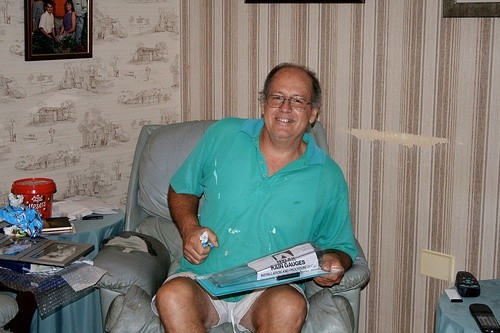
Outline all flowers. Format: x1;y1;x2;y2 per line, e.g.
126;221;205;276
0;193;44;239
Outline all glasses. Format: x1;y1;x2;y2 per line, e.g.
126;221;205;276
265;94;313;111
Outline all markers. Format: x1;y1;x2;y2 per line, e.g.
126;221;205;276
82;216;104;220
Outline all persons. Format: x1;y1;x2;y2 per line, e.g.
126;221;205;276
33;0;87;53
151;64;360;333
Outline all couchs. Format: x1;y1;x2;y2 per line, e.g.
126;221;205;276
91;120;370;333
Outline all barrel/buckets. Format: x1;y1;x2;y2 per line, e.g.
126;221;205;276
10;177;56;218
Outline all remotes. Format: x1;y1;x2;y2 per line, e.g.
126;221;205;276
456;271;480;298
470;304;500;333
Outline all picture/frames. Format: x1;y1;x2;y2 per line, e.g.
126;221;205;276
443;0;500;17
24;0;93;61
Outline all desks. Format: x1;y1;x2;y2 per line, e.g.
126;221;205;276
31;209;126;333
434;279;500;333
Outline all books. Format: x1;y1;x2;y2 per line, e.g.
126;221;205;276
41;216;73;235
